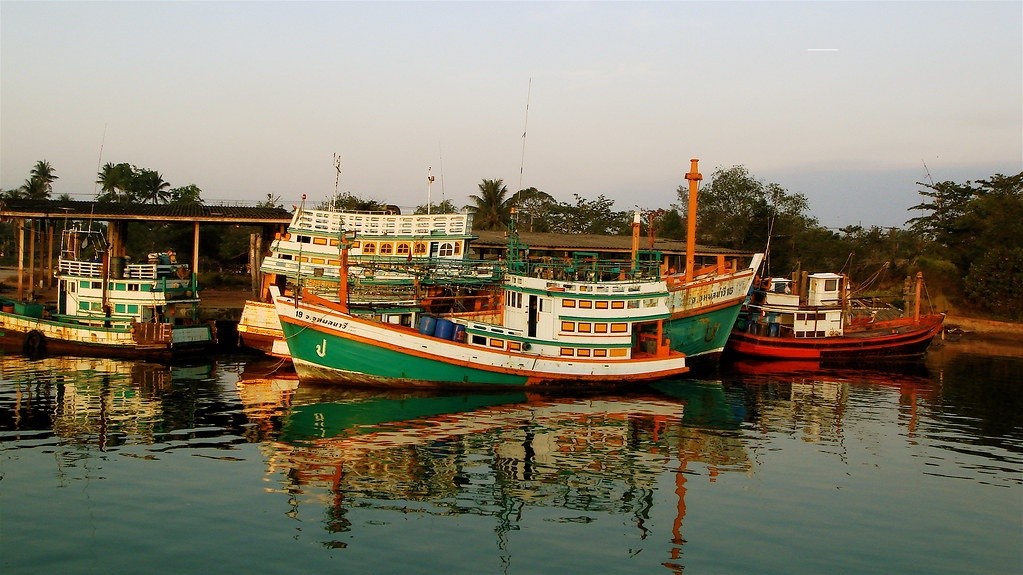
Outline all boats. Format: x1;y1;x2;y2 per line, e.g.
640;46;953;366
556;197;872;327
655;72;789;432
0;122;219;358
262;374;683;535
269;213;690;388
453;157;764;368
727;248;950;374
235;152;513;361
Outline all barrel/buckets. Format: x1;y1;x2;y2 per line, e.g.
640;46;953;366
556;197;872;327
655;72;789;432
768;323;780;338
419;314;437;336
111;256;125;280
434;318;454;340
756;322;769;337
3;303;15;314
451;324;465;344
744;320;756;336
